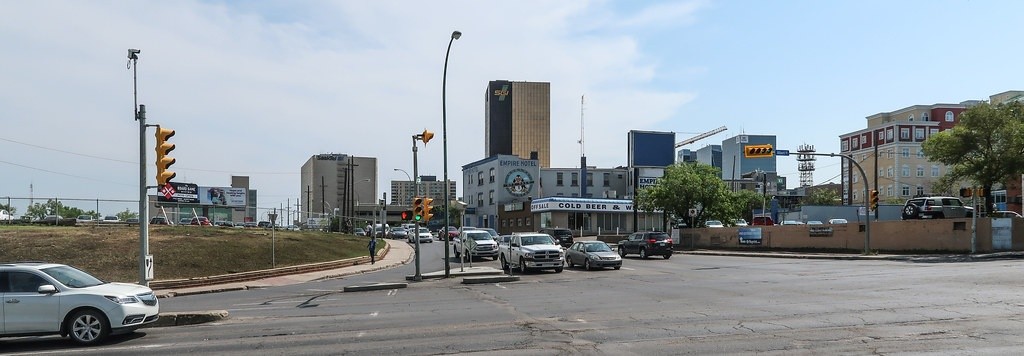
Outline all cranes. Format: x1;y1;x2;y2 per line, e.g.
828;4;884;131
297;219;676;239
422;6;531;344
675;126;727;150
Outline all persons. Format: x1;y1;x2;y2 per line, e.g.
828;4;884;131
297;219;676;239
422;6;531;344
991;202;997;211
365;222;372;237
472;234;477;240
587;245;593;252
422;229;427;233
596;245;604;251
367;236;375;265
39;278;49;285
539;238;545;243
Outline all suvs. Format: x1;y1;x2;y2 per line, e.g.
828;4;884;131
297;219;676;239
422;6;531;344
901;193;973;219
538;228;574;248
453;230;564;273
618;231;674;260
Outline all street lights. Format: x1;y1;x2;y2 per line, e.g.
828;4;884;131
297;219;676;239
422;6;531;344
394;168;412;206
441;30;462;276
323;179;371;218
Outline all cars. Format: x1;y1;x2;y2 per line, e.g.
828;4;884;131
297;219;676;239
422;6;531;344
0;207;299;229
352;223;476;243
704;215;852;228
565;241;623;270
0;262;161;346
993;211;1023;217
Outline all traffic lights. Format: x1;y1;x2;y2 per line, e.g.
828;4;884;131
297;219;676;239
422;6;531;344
744;145;773;157
402;197;434;223
155;127;176;186
